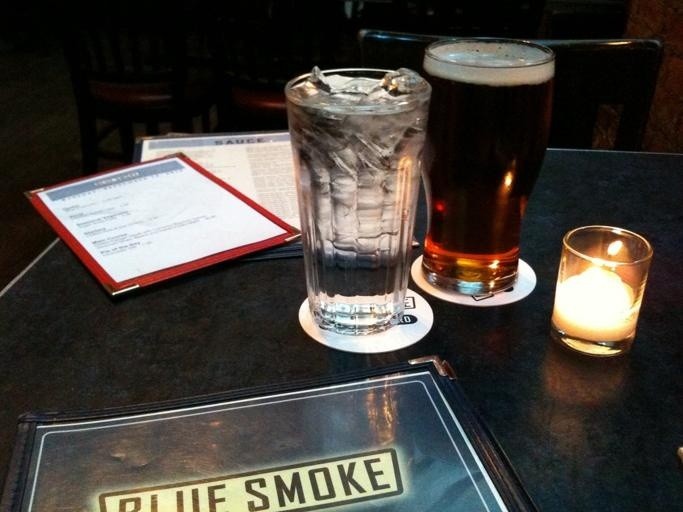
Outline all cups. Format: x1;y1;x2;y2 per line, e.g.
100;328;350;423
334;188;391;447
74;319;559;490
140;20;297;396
418;37;555;297
550;226;653;356
288;65;430;336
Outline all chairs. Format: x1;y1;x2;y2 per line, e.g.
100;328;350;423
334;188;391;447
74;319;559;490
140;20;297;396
358;31;665;155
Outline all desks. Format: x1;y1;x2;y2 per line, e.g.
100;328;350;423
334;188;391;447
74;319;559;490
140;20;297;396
2;148;683;510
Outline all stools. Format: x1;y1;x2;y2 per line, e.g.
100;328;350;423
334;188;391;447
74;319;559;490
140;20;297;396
82;78;291;172
